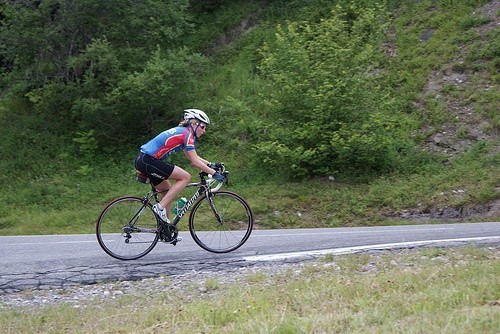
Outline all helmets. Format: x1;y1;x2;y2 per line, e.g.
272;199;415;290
184;109;210;125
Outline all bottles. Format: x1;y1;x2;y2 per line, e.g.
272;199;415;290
172;197;188;214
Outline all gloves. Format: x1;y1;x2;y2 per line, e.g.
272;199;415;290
212;172;225;183
208;162;224;171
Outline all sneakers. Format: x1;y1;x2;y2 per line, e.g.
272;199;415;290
152;203;170;223
171;233;182;240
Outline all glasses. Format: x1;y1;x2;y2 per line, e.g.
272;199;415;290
195;122;206;129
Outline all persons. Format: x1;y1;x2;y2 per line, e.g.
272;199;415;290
134;109;226;244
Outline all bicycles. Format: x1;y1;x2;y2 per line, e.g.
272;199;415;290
96;161;254;261
